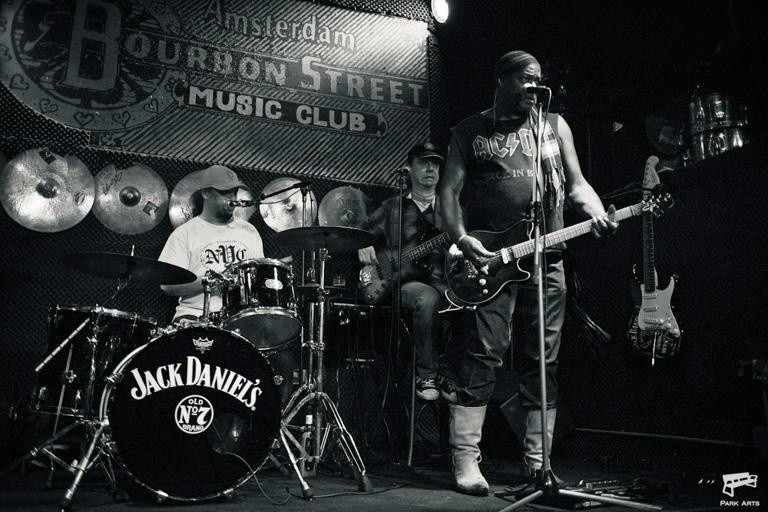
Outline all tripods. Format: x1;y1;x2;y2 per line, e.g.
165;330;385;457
381;189;436;489
496;105;661;511
262;193;371;495
19;326;129;512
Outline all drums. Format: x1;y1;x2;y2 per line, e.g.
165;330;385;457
222;258;302;353
33;306;167;419
682;85;756;166
100;321;282;503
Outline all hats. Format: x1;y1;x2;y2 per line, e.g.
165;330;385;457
200;165;249;194
408;141;445;166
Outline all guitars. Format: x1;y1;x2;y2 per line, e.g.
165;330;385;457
627;155;683;365
345;230;450;304
443;184;673;304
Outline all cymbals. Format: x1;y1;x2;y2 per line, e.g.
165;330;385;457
317;186;375;231
63;253;197;285
170;173;255;230
275;227;378;249
260;176;318;234
94;163;168;235
1;147;95;233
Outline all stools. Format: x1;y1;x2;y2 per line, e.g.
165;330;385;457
381;307;449;465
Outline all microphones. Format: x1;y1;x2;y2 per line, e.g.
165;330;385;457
519;82;550;101
226;198;266;212
395;163;414;184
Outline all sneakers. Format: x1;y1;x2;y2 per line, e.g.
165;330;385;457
416;373;458;403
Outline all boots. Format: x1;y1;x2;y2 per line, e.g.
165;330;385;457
522;405;564;483
447;403;491;494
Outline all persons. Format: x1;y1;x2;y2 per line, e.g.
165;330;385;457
156;165;267;326
440;51;620;496
355;144;459;404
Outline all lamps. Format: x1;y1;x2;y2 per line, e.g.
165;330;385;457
432;0;449;23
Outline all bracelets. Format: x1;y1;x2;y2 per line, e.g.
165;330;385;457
455;234;466;247
